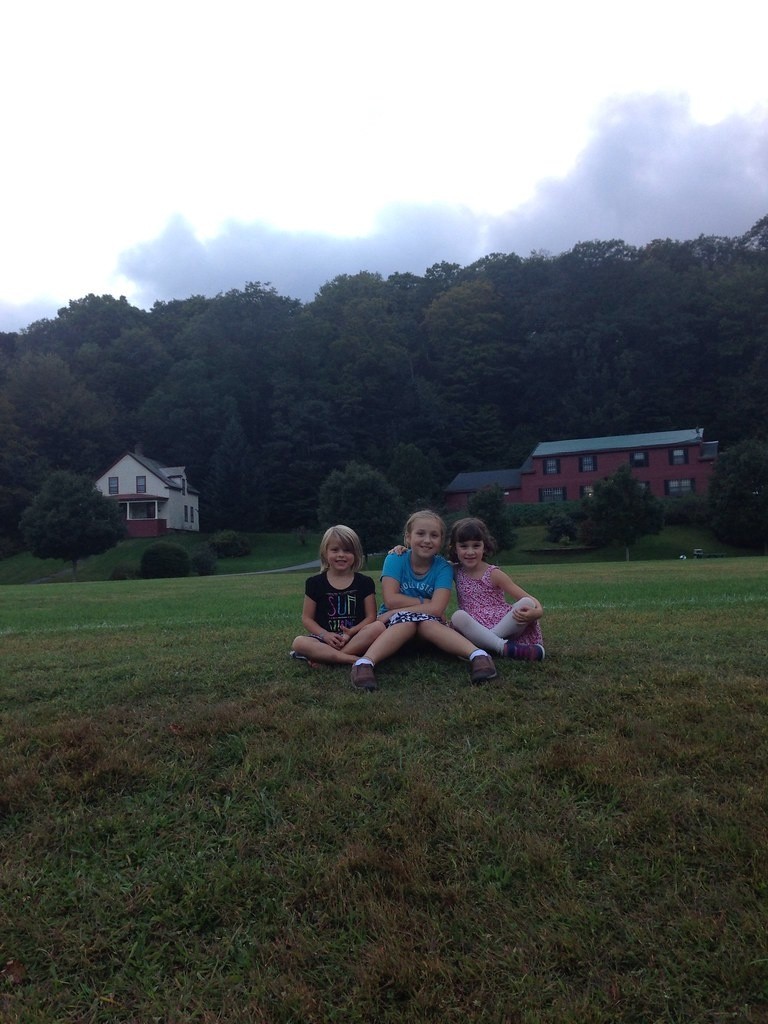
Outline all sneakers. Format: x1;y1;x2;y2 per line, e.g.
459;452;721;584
470;654;499;684
505;642;546;661
351;663;378;692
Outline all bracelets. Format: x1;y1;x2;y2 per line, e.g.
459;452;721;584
319;632;324;638
419;597;423;604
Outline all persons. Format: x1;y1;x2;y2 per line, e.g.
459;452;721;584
388;518;545;662
350;509;496;691
289;525;386;669
